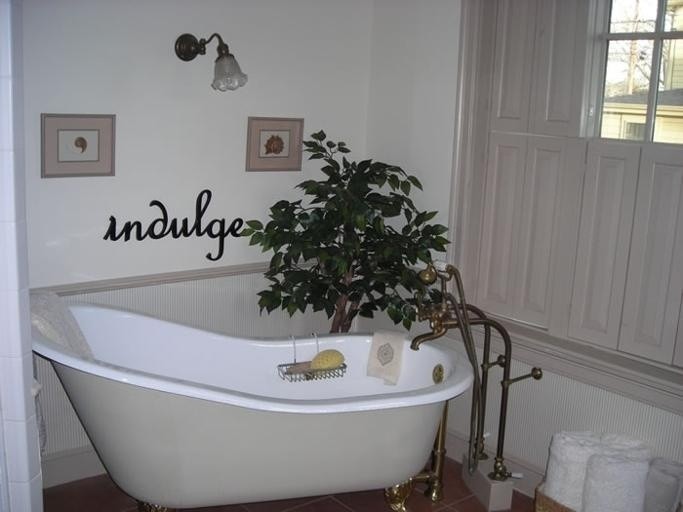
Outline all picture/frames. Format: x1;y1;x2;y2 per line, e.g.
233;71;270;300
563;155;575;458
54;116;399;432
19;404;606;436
40;114;116;178
246;116;305;171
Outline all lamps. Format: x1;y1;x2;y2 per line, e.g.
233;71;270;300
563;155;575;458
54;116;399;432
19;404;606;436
174;32;248;92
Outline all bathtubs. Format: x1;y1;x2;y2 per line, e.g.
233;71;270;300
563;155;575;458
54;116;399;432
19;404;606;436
32;303;474;512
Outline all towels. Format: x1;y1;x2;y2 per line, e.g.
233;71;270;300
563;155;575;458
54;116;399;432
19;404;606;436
366;331;405;385
541;430;683;511
29;290;95;450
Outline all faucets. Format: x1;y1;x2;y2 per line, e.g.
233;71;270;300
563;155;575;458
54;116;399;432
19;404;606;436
410;327;447;350
